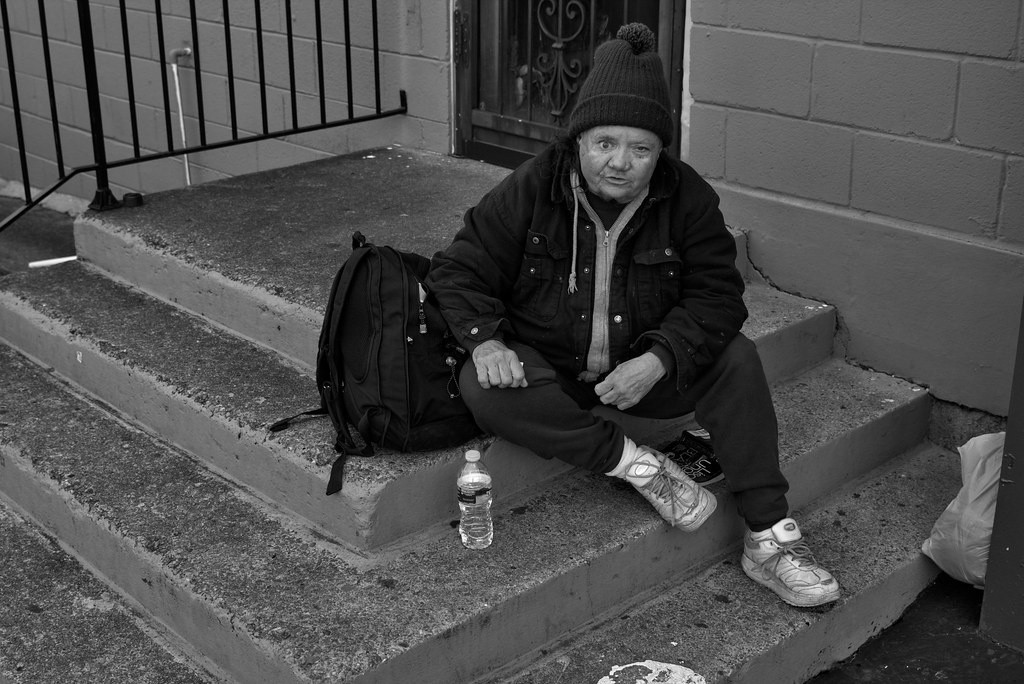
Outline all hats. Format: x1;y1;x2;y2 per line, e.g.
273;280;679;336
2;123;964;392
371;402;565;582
568;22;674;146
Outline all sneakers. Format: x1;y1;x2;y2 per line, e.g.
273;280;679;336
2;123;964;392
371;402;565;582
741;518;840;607
624;445;717;533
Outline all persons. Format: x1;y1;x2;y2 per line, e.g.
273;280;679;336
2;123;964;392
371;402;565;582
426;22;840;608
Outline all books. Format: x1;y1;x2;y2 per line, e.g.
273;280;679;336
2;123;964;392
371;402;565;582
660;427;726;487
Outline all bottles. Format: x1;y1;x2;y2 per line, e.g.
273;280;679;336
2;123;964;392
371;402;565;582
457;450;495;549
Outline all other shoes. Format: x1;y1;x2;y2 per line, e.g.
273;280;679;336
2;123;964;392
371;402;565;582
660;427;725;487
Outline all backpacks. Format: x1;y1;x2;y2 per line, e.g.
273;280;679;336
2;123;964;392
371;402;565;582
316;231;482;457
